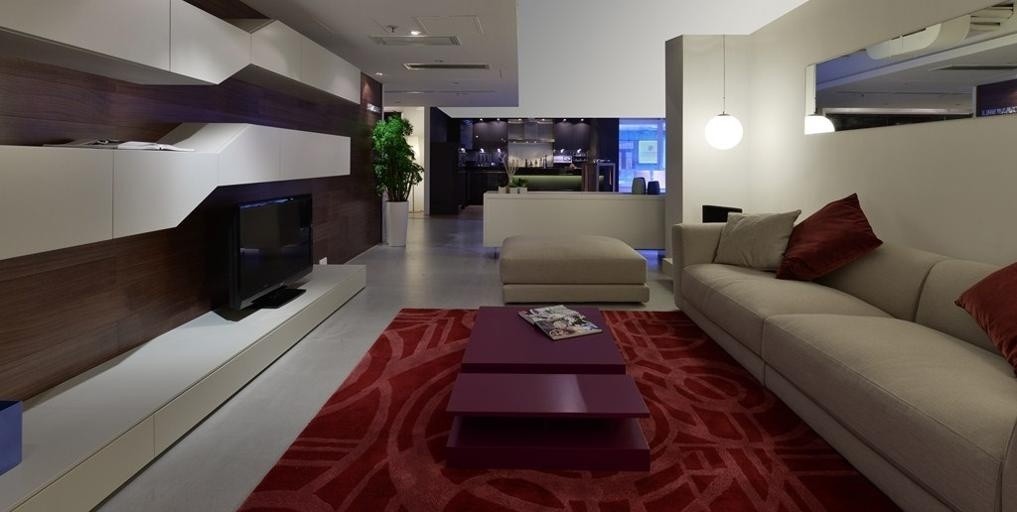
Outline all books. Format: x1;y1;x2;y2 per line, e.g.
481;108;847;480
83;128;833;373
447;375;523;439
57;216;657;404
518;305;603;340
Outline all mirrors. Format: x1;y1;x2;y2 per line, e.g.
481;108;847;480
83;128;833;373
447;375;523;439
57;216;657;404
799;0;1016;138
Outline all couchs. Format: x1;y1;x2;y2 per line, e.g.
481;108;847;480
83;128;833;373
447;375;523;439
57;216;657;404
499;234;650;304
669;217;1017;510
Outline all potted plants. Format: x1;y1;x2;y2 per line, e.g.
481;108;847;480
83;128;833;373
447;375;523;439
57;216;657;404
366;110;424;249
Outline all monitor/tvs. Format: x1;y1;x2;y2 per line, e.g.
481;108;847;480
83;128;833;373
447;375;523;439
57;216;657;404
226;194;314;310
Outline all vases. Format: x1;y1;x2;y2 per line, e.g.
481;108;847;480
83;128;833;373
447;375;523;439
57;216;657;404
646;180;662;196
630;175;648;195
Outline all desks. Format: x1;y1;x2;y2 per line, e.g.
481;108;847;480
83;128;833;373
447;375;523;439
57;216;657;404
0;264;367;511
481;190;666;262
444;305;652;474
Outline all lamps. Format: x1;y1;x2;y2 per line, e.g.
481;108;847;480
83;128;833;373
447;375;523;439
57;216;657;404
803;85;837;135
705;34;745;154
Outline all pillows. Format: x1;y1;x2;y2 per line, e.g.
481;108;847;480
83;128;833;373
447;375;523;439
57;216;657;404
774;191;886;282
952;260;1016;379
711;208;802;272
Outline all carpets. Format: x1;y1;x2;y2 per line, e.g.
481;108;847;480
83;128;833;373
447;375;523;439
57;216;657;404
233;305;902;510
